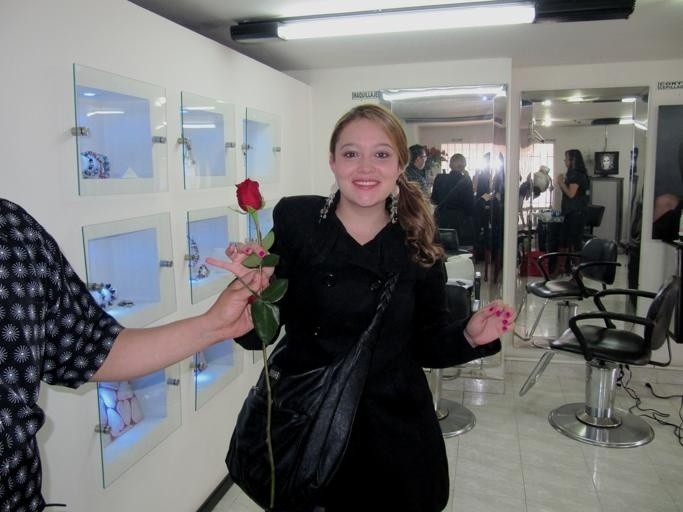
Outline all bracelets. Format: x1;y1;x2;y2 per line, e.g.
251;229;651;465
187;238;209;281
92;283;116;307
86;286;105;308
78;152;110;179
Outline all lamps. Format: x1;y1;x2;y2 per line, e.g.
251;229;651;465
229;0;636;44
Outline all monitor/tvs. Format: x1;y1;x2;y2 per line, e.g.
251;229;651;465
595;152;618;178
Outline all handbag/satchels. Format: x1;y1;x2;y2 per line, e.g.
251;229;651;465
226;342;368;511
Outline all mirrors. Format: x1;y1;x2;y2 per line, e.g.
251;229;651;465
380;83;506;310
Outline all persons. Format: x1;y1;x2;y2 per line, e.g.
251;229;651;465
0;197;272;512
599;154;613;170
203;103;518;511
555;148;590;255
402;142;505;268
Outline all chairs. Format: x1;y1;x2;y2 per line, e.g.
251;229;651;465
423;366;477;438
507;236;622;343
574;204;605;249
519;274;682;448
437;229;476;259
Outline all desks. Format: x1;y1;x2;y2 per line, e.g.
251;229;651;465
537;218;566;270
590;177;623;249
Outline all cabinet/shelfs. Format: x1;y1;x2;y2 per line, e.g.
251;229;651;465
100;362;170;460
190;218;233;288
76;87;158;179
179;108;227;178
86;226;162;318
194;343;236;385
247;204;278;259
244;117;276;180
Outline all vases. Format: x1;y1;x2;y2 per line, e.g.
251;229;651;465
424;169;436;185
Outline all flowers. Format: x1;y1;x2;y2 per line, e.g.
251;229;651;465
420;143;450;171
230;177;292;508
